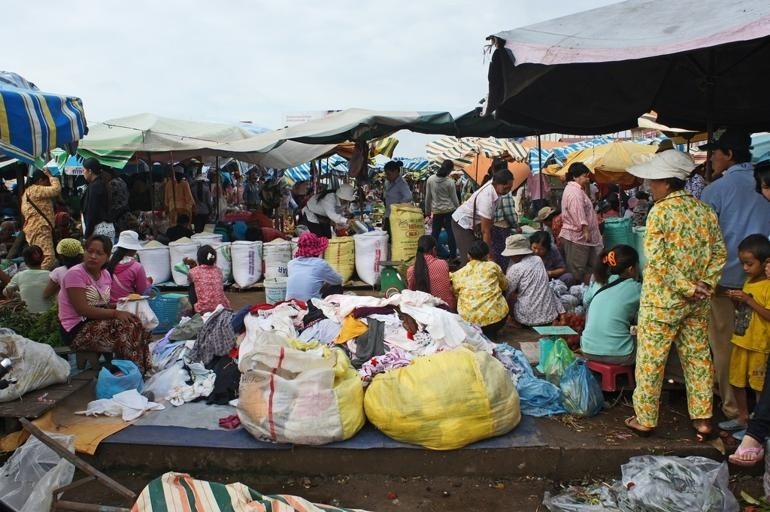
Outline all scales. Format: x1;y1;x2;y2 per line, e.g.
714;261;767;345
376;260;408;292
532;325;578;378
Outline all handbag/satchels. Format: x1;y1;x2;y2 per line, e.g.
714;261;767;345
474;224;482;241
144;286;180;334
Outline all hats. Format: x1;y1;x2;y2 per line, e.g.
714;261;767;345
625;148;696;181
293;233;329;258
56;237;84;258
337;184;356;202
113;230;144;251
655;139;674;154
698;128;752;150
532;206;556;223
500;234;533;257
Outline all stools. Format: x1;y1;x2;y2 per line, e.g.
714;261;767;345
584;361;633;393
75;350;114;370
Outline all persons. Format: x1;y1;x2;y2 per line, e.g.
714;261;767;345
0;125;770;469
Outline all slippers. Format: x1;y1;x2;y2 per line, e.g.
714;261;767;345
718;418;766;467
625;416;651;438
691;419;714;441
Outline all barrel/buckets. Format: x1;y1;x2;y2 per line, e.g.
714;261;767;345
263;276;288;306
